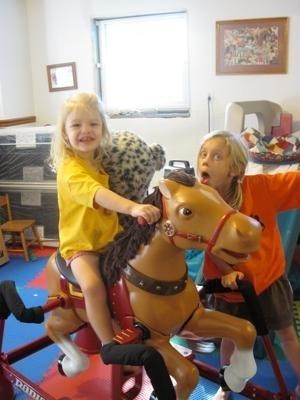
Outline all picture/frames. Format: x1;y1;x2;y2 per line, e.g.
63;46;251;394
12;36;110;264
215;16;290;77
46;62;78;92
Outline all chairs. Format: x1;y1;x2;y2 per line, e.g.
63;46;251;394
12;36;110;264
0;194;44;262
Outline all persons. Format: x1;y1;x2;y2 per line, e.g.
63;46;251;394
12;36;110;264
42;91;163;373
195;130;300;400
